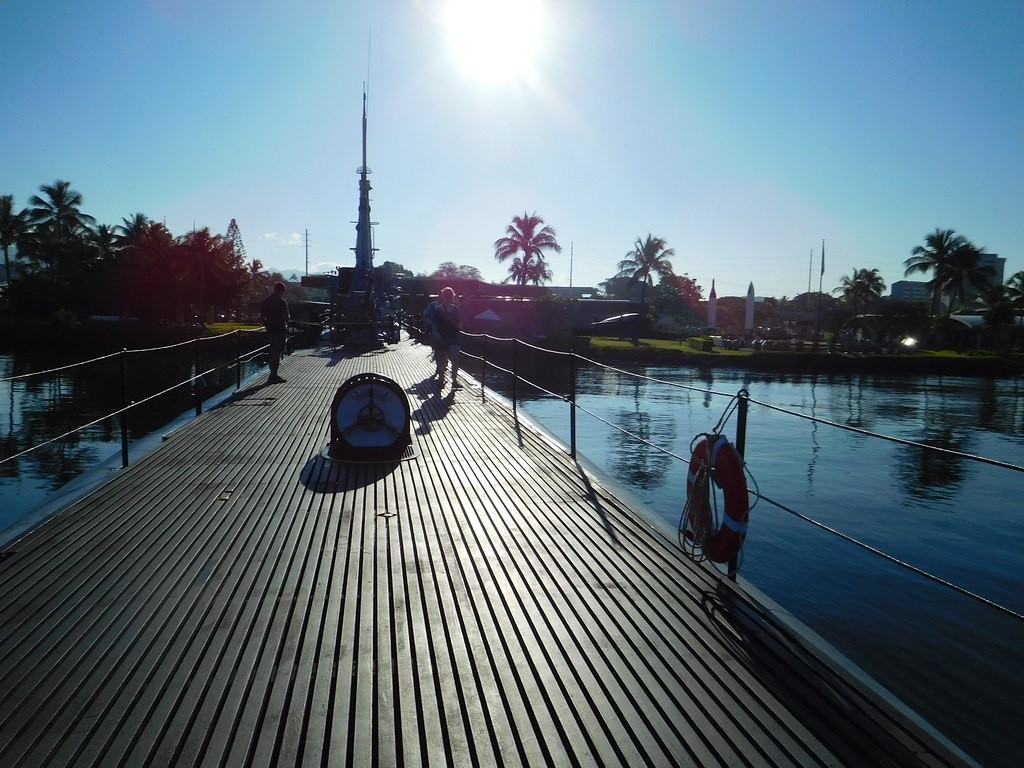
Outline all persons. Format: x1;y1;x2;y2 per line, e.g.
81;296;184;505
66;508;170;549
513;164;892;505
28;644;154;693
424;287;463;389
262;282;291;384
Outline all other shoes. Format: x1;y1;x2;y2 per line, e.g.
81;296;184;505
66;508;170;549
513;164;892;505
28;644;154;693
437;380;444;389
452;382;463;388
268;375;286;384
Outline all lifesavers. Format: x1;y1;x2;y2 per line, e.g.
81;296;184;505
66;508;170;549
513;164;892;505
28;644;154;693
685;434;751;563
